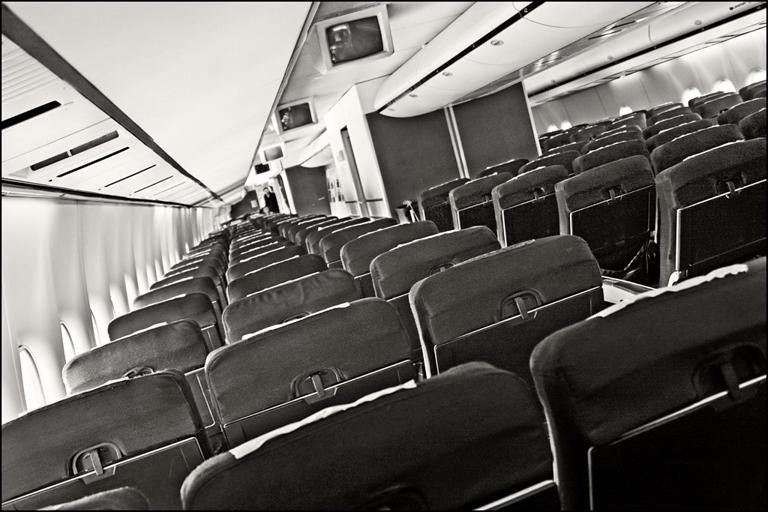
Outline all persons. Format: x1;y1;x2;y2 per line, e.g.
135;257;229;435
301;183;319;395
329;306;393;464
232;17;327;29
261;187;280;214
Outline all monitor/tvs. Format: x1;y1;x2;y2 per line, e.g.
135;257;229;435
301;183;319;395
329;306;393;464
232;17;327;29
253;163;271;176
258;141;287;166
273;98;319;136
316;3;394;75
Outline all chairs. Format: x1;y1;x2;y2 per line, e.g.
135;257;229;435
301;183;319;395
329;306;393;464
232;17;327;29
553;153;660;289
570;124;599;144
648;102;683;118
172;235;223;265
480;158;529;180
1;367;215;511
645;118;715;153
717;96;767;124
221;269;362;345
692;92;732;106
539;130;562;148
227;222;274;238
542;131;574;147
449;172;513;237
179;360;561;512
149;266;227;309
257;211;288;229
739;79;767;102
61;318;209;399
538;139;588;153
318;216;398;270
204;296;412;445
416;175;472;231
647;107;692;128
107;292;224;351
688;90;723;107
607;114;646;130
518;150;580;171
305;216;370;255
227;240;293;268
643;112;703;140
224;244;308;285
694;94;743;119
295;215;351;247
36;485;152;511
339;219;438;278
225;253;328;306
593;124;642;138
288;215;328;244
490;163;569;248
739;107;768;138
580;130;640;155
614;113;647;118
650;123;744;175
529;254;767;510
368;225;502;366
408;233;603;376
132;276;225;344
570;138;648;174
654;136;768;289
